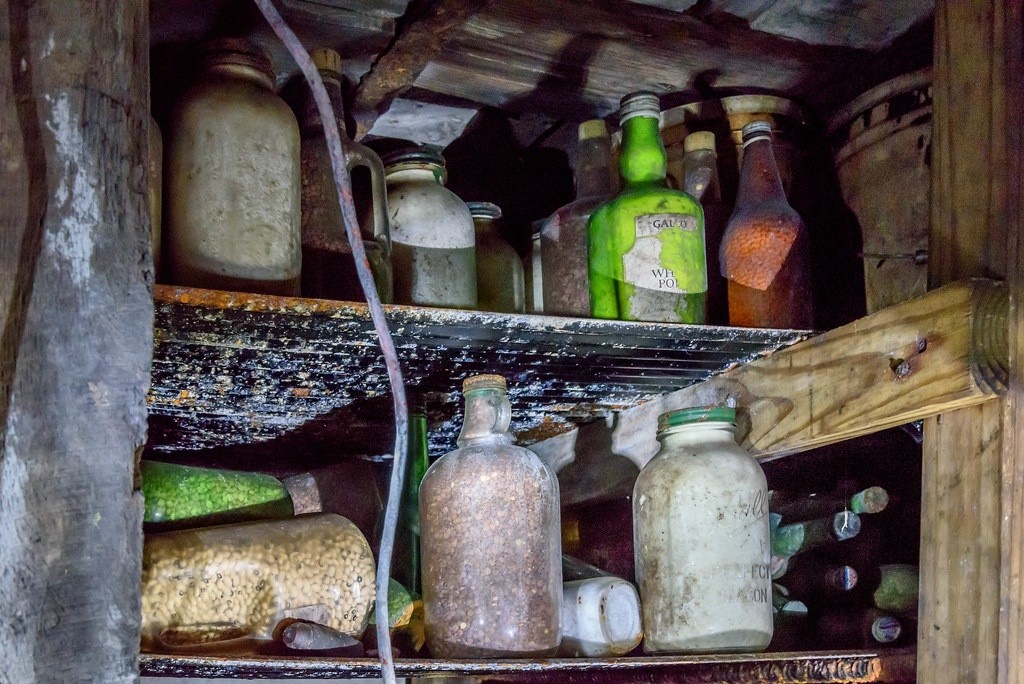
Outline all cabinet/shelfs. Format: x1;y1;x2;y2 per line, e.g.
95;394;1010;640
0;0;1024;684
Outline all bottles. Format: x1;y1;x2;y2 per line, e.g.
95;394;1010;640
134;37;904;656
